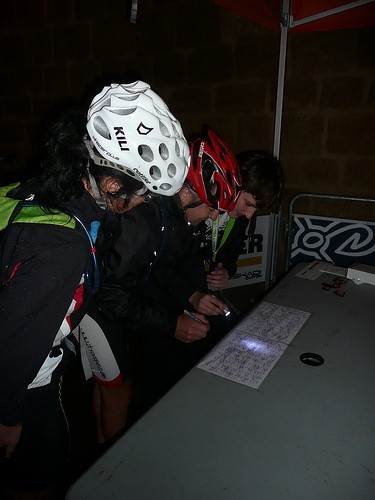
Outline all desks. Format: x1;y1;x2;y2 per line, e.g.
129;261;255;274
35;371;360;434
66;260;375;500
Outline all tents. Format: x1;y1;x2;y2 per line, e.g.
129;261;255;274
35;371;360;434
211;1;375;291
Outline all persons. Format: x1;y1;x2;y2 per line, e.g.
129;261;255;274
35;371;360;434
181;150;284;363
87;123;244;462
1;80;191;500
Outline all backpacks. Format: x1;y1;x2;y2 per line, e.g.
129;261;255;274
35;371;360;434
0;173;100;288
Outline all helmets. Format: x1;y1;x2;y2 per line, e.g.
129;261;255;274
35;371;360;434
81;79;192;195
184;127;246;214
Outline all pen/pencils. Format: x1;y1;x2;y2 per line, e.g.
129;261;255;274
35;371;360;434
183;309;209;327
